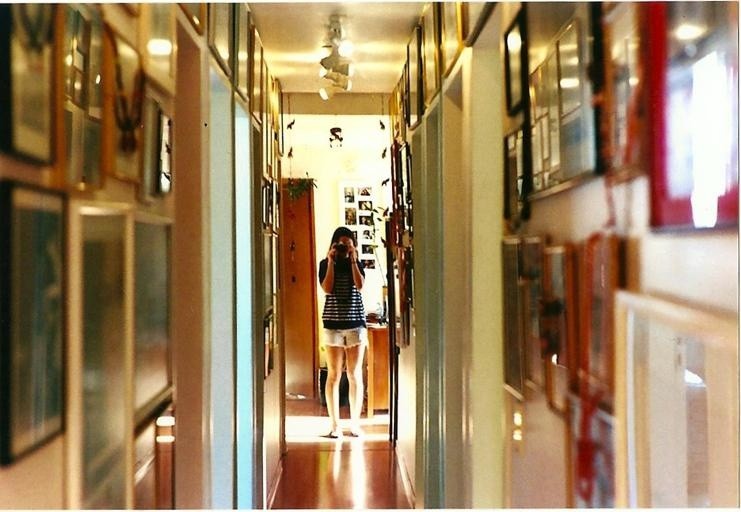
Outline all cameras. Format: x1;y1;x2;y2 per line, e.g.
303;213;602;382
332;242;349;260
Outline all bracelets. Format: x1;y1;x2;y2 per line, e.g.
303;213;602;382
351;260;357;264
330;260;337;264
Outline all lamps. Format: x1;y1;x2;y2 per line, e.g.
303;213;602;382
318;13;355;102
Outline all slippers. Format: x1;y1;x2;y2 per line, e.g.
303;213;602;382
351;431;360;437
329;431;338;438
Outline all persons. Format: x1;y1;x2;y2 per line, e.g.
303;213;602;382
319;226;373;437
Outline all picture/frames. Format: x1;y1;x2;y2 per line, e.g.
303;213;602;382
394;2;737;507
4;3;283;508
